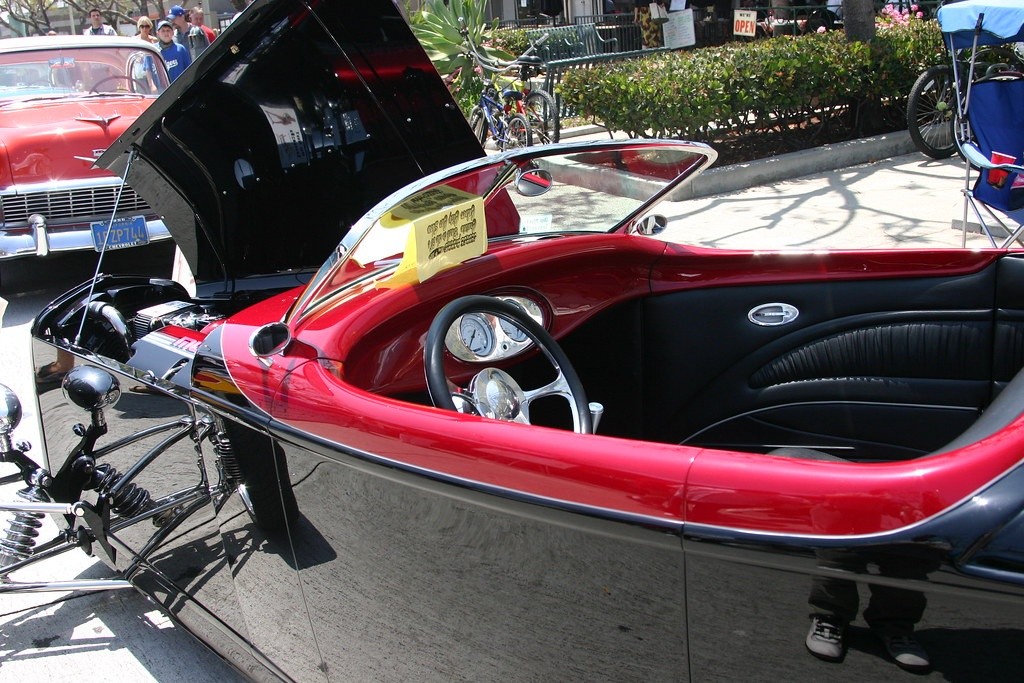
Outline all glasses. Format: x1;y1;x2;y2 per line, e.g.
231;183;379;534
139;25;150;29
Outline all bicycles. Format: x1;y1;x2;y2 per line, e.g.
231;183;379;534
453;15;560;153
905;47;1024;159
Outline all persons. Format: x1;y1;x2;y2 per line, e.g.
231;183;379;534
606;0;616;13
48;8;121;79
803;0;843;33
167;4;210;61
131;16;158;94
143;21;190;95
188;6;215;44
633;0;665;50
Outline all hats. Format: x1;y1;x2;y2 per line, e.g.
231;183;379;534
166;5;185;19
157;21;174;31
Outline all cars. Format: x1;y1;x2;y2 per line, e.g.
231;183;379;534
0;0;1024;683
0;35;176;295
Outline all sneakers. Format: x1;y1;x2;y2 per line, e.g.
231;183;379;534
806;617;846;658
884;633;931;667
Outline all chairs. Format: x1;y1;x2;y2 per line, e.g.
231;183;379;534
951;71;1024;248
651;257;1023;463
